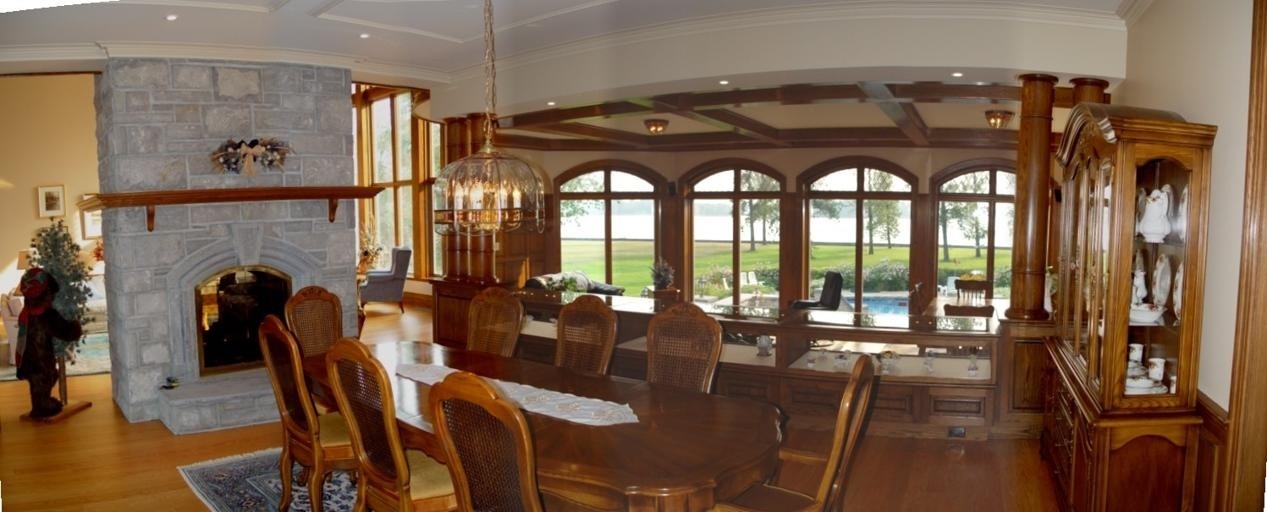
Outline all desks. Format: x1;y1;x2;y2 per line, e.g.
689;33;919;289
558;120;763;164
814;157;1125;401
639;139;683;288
921;293;1011;323
283;335;785;512
790;270;845;310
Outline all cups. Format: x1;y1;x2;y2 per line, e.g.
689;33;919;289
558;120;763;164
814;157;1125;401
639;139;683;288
654;298;664;311
605;296;612;306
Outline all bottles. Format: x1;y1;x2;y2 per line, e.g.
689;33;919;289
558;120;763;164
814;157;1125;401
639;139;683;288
756;334;772;356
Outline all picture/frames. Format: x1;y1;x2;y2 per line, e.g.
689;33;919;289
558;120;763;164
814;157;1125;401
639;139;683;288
80;192;102;240
36;184;66;220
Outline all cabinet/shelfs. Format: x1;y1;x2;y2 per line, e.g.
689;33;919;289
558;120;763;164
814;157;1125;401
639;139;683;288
1044;347;1204;511
1049;102;1220;416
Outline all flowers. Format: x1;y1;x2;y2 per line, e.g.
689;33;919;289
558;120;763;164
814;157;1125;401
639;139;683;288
651;259;674;287
210;136;293;176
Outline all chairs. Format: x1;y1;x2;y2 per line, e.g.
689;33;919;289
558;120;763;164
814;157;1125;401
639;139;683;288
427;368;547;512
945;305;997;318
283;285;347;415
458;285;525;356
707;352;878;510
247;315;358;511
360;245;412;313
326;338;459;512
953;278;993;298
553;293;620;376
646;302;722;394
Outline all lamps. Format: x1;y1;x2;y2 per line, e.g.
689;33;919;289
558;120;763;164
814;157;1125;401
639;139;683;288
424;1;553;242
643;119;669;136
985;109;1014;129
15;247;43;272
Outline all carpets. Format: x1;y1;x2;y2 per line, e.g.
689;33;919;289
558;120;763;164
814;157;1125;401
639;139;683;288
0;326;114;382
175;447;366;510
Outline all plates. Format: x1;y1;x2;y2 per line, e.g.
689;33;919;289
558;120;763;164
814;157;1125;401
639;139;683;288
1122;184;1188;397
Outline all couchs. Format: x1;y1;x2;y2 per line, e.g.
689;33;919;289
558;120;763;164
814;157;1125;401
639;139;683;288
528;268;624;296
1;259;24;365
